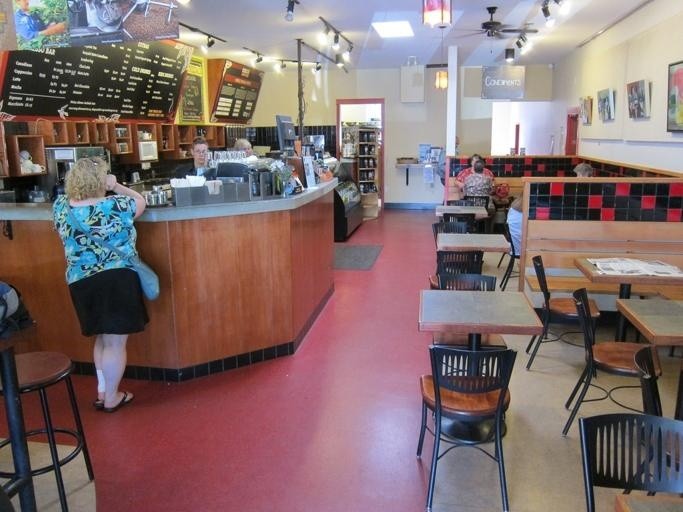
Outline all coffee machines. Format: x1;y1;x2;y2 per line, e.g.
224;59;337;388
38;146;104;199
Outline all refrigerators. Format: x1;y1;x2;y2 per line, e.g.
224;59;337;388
341;125;379;218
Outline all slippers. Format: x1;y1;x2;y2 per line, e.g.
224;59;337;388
93;391;135;413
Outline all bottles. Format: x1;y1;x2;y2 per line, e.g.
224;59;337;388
144;186;168;205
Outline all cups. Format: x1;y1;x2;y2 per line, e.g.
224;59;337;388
203;150;247;170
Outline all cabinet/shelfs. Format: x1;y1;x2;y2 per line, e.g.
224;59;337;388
357;126;378;207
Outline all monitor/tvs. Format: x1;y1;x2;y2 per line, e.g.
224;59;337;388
276;115;296;151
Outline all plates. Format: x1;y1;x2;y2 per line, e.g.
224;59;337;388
92;8;121;33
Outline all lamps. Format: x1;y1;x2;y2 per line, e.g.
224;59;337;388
205;35;216;48
422;1;450;90
255;52;264;64
331;33;354;68
285;1;299;23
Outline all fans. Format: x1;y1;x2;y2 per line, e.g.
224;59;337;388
456;6;538;40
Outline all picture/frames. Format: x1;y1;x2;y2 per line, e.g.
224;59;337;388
666;60;683;133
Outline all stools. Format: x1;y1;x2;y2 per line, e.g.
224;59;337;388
1;345;93;512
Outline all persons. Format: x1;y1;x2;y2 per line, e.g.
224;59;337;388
506;189;523;256
574;162;594;177
51;153;149;415
173;137;216;181
462;159;496;216
629;87;640;119
604;96;609;120
14;0;65;44
233;138;253;158
453;153;496;192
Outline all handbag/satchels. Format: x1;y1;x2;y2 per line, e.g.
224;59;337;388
495;183;510;197
130;253;160;300
